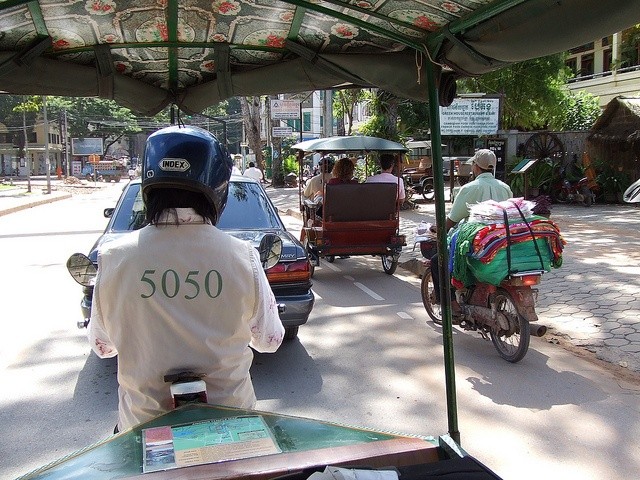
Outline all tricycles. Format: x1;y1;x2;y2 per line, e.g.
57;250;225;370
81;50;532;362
404;157;434;200
291;135;409;274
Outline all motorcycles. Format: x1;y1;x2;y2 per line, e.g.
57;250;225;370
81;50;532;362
552;170;592;206
413;220;548;363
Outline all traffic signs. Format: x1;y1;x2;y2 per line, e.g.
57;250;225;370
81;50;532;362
272;127;292;138
270;100;300;120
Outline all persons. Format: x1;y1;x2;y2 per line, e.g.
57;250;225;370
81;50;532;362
128;167;136;176
365;154;406;205
429;147;514;324
303;157;334;220
84;126;287;435
243;162;264;184
230;165;242;178
330;158;359;184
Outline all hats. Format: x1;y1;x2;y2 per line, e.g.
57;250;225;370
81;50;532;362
466;149;496;170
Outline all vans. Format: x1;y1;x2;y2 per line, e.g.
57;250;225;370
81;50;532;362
405;141;453;181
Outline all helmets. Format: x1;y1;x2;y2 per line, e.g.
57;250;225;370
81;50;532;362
141;124;230;225
319;158;335;174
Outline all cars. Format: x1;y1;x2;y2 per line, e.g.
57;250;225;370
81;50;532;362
77;175;314;339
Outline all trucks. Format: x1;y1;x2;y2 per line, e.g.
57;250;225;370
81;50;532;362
83;161;121;182
